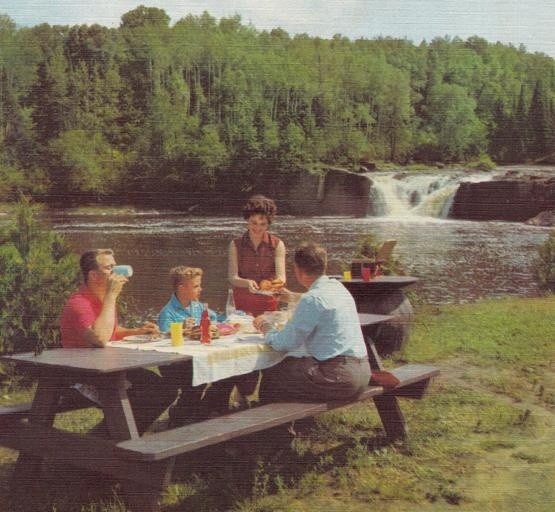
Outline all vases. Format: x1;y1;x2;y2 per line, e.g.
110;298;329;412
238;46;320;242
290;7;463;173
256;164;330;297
318;274;417;358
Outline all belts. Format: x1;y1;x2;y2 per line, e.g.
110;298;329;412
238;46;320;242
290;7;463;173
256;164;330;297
332;357;372;364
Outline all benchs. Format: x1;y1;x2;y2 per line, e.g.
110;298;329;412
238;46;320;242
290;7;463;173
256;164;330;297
1;398;97;421
114;364;440;512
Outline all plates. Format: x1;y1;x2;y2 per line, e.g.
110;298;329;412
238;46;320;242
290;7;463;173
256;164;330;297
123;334;163;343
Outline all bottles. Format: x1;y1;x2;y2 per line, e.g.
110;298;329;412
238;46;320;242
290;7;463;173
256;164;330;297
200;302;213;345
225;288;236;320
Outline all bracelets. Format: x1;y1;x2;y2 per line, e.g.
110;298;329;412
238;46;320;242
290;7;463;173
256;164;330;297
258;320;272;333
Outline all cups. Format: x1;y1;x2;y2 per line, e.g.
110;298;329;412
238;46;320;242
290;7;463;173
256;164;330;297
344;271;351;281
169;322;184;349
361;268;371;281
111;264;134;279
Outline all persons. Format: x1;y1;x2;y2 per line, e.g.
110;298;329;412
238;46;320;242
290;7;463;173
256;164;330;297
227;194;286;407
60;249;178;441
252;241;372;405
156;265;234;420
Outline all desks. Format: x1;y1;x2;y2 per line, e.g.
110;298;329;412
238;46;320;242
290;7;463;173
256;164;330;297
0;313;395;512
